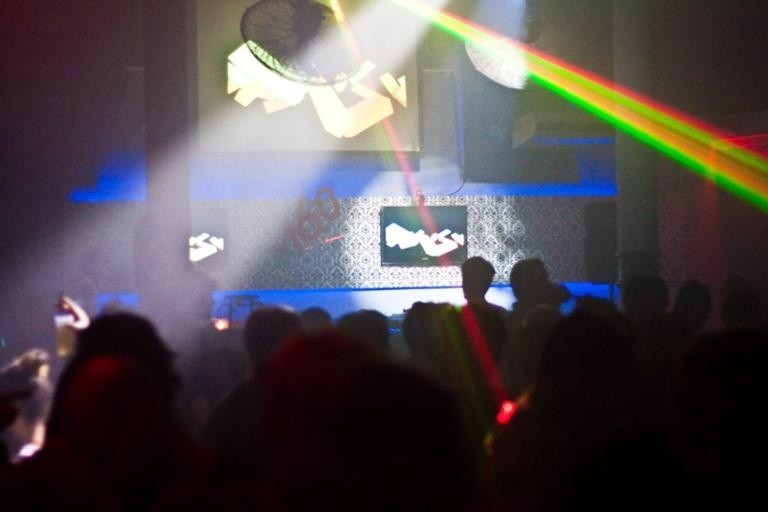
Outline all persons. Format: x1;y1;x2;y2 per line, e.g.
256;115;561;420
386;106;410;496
0;249;768;512
